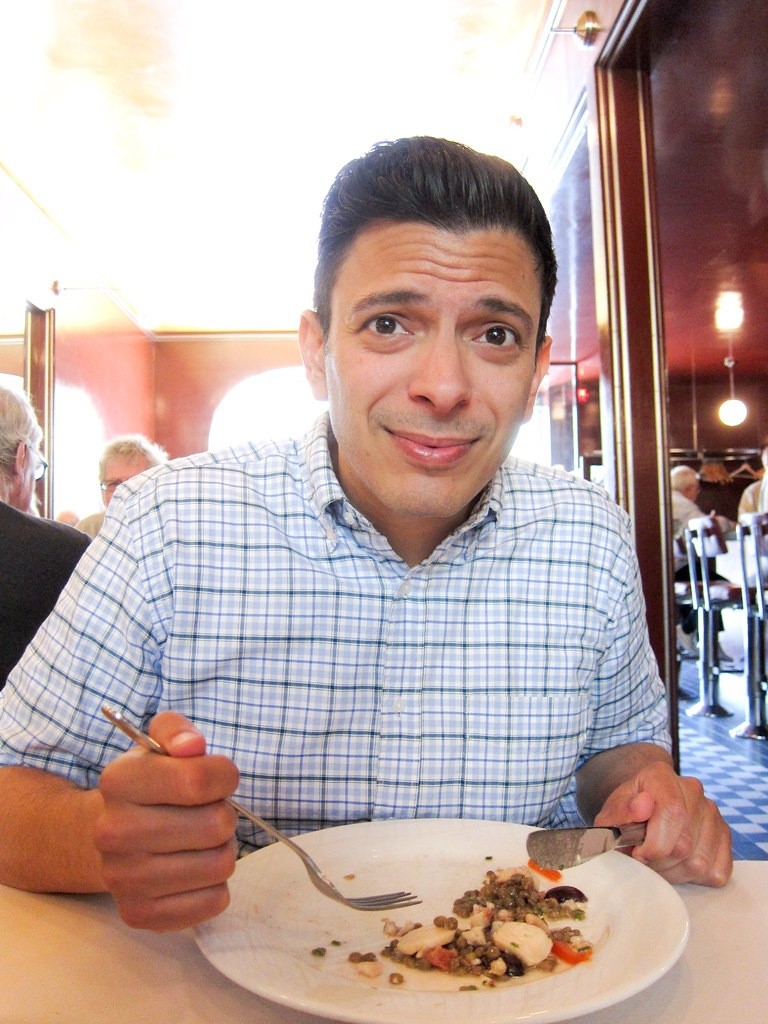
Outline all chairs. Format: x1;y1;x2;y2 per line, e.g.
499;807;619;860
674;512;768;743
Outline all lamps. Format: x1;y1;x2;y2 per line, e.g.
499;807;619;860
718;331;748;426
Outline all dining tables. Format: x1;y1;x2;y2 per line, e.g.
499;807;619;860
2;858;768;1024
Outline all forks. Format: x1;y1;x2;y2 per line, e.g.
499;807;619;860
95;702;424;913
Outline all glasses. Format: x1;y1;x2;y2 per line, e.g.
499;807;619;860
26;444;47;481
100;479;126;491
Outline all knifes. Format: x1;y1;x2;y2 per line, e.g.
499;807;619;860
524;820;645;875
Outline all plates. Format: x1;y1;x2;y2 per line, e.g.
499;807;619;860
186;816;691;1024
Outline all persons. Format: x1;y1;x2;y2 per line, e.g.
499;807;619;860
737;438;768;524
73;433;170;539
0;384;90;695
0;137;733;931
670;465;732;661
